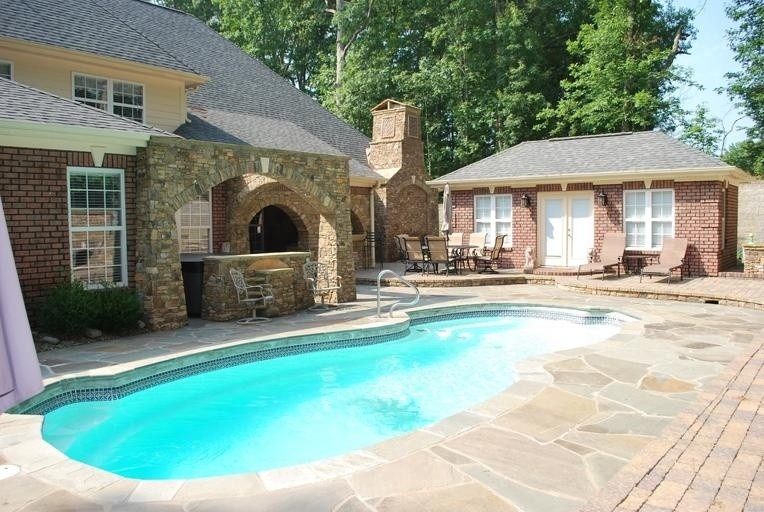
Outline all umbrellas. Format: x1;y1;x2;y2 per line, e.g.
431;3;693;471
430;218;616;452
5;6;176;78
0;192;46;418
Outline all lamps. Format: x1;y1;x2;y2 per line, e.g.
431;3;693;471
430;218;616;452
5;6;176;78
521;194;529;207
597;192;607;206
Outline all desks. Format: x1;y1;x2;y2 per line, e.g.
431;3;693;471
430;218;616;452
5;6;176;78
625;255;650;277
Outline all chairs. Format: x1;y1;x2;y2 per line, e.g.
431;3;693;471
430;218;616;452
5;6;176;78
303;262;343;311
577;231;627;281
640;237;688;285
395;232;508;277
230;267;274;326
